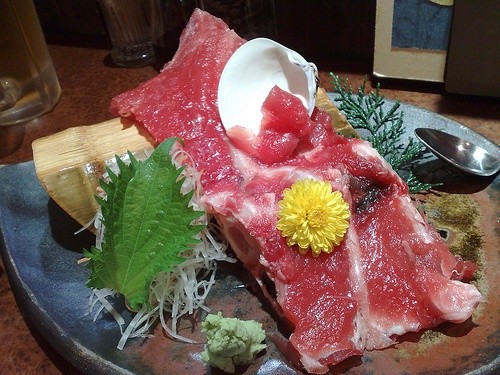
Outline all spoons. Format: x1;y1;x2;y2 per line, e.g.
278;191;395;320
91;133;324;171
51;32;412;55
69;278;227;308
414;128;500;176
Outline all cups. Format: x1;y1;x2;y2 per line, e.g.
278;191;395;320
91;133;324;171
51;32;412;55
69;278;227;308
102;0;164;68
0;0;62;126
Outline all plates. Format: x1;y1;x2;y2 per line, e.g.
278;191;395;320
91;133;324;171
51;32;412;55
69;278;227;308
0;92;500;375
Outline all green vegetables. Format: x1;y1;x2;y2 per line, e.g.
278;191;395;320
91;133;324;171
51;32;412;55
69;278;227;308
81;138;206;315
